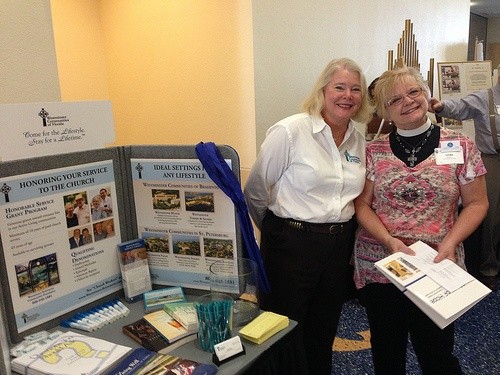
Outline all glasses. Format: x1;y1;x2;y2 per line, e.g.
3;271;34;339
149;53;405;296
386;87;423;107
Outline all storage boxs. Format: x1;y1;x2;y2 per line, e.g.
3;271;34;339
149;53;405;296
116;239;152;303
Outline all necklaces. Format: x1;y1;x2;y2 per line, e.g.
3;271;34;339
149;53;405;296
394;123;433;166
334;133;345;142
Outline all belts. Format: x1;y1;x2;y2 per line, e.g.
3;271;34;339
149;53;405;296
280;220;354;235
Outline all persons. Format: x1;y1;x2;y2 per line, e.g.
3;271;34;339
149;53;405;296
244;58;368;375
429;63;500;291
66;189;141;265
352;68;489;375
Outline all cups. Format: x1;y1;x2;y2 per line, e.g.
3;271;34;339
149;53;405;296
192;293;234;352
209;256;261;328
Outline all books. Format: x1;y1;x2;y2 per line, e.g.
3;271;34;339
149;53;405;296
10;286;219;375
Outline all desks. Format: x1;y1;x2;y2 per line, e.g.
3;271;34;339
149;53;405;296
7;288;298;375
